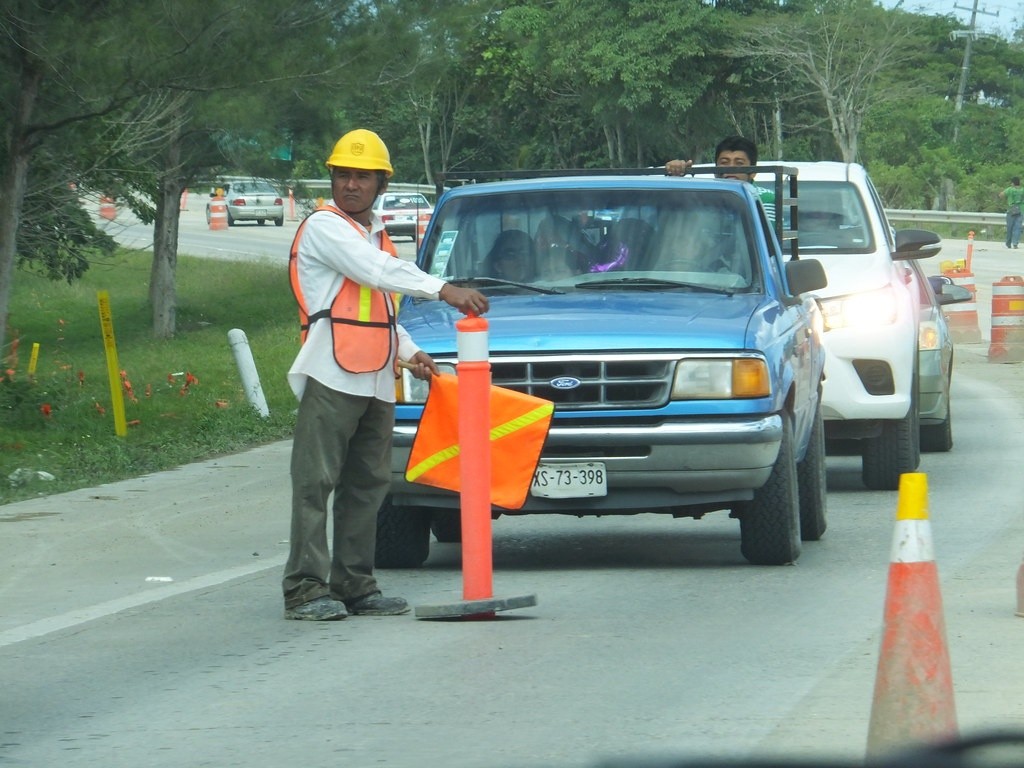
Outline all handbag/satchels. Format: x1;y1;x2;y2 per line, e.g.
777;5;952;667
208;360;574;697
1008;204;1020;215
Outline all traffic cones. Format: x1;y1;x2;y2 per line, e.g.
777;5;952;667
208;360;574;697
863;471;961;768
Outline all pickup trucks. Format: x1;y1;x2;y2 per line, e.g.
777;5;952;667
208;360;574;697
376;159;828;571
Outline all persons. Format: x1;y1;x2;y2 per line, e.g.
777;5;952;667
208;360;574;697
281;129;489;620
999;177;1024;249
537;247;582;282
483;230;536;282
666;136;783;232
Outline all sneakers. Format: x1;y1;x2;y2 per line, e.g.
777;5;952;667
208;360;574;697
284;597;347;621
346;590;410;615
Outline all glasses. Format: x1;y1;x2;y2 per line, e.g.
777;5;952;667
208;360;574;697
494;247;534;260
541;239;572;258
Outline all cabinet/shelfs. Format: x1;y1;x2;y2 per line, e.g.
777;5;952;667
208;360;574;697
434;165;800;261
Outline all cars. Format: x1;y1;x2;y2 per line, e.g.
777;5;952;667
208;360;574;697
372;192;433;242
205;180;285;227
824;215;974;451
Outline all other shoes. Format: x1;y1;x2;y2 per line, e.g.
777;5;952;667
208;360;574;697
1014;245;1018;249
1007;242;1011;248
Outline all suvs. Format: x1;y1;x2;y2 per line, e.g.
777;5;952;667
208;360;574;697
616;156;944;492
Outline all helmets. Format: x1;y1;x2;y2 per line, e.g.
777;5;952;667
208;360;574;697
325;129;394;180
